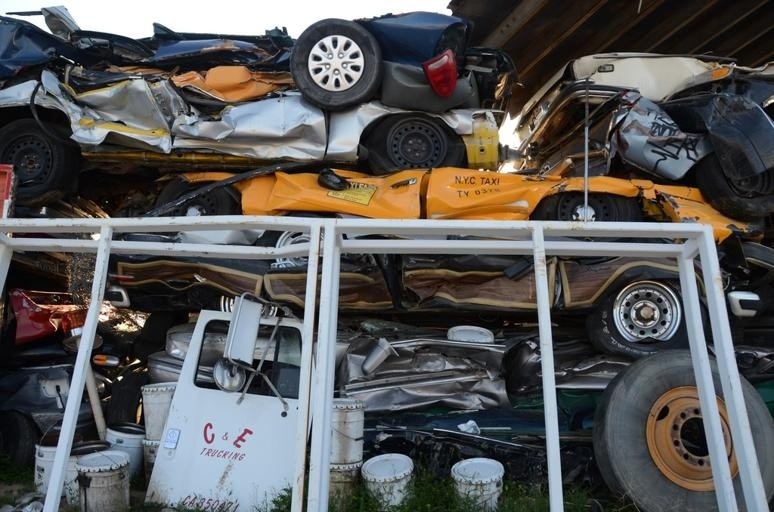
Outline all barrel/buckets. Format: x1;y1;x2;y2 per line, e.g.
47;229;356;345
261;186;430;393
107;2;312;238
362;452;412;510
332;397;365;463
65;441;112;505
107;427;144;478
76;449;129;512
450;457;505;512
142;382;176;441
34;418;60;496
141;438;159;486
330;469;356;511
